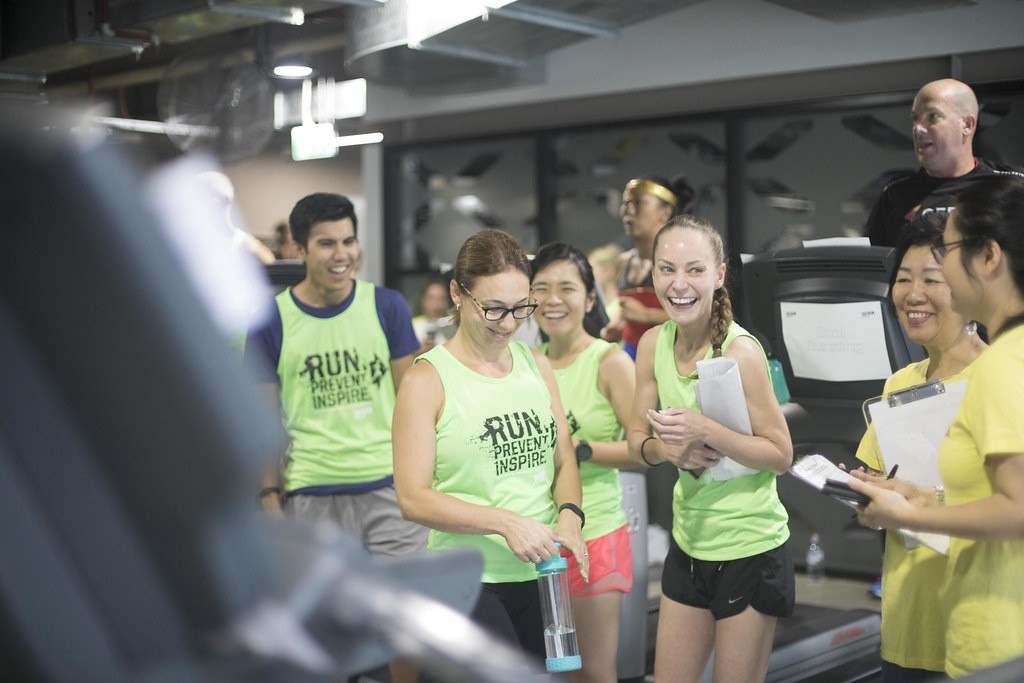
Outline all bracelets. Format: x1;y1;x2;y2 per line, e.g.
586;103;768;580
933;485;945;508
259;486;281;499
558;503;585;529
640;436;664;468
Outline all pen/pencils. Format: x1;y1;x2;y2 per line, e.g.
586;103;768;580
888;464;899;479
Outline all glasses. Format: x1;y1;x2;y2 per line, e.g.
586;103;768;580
929;236;985;263
456;281;540;321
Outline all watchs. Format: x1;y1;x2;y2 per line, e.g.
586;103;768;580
576;439;593;461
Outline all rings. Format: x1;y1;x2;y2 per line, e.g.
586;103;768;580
584;553;588;556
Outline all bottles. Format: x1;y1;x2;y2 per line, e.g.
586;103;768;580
805;532;826;586
766;351;793;404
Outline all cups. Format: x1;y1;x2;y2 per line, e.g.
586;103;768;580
535;542;583;673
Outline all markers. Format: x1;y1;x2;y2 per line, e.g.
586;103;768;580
823;478;870;504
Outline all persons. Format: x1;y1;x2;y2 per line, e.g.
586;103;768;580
600;173;680;365
392;228;590;682
409;243;628;360
241;191;430;563
837;211;988;682
846;177;1024;683
625;213;794;683
866;78;1024;252
526;242;642;683
